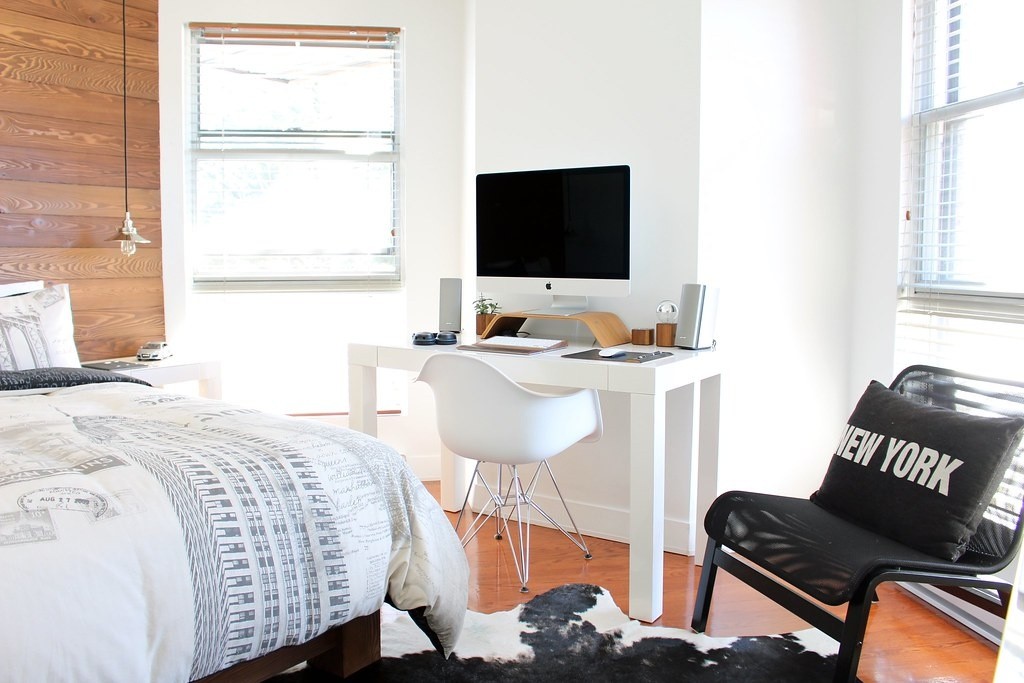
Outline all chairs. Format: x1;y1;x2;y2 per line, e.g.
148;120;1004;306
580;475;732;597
413;352;603;595
692;362;1023;683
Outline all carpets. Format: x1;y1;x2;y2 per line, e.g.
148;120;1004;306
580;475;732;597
259;583;864;682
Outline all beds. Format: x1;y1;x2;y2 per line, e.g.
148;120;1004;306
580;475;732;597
0;280;470;683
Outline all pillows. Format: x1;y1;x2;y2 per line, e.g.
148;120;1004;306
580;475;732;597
1;283;83;371
810;381;1023;563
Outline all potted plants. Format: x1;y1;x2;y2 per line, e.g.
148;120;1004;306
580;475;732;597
474;292;499;336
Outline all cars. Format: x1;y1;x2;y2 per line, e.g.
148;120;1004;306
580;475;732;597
135;342;173;361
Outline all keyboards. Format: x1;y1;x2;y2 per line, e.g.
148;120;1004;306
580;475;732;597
474;335;568;353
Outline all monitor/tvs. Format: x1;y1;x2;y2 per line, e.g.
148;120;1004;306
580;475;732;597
476;164;632;316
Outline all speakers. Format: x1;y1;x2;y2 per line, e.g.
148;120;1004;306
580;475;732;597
439;278;462;334
674;284;718;349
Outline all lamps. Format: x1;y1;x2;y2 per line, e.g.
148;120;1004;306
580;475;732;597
103;1;153;255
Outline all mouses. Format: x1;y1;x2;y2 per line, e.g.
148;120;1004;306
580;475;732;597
598;347;626;358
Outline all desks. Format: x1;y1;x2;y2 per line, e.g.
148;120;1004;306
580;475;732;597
347;331;720;625
